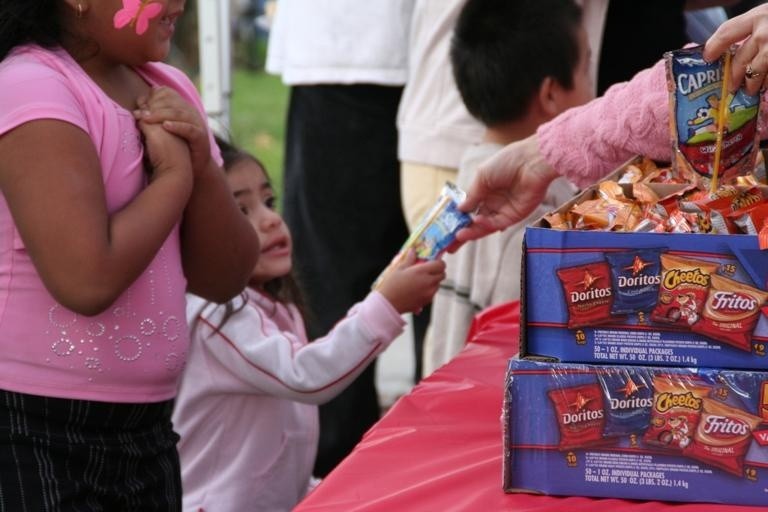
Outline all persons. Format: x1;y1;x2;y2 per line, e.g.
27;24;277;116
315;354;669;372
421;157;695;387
263;0;416;491
420;1;594;384
1;1;258;512
447;3;768;255
396;1;611;253
168;148;447;512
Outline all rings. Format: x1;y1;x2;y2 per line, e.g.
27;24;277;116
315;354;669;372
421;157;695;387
744;66;761;80
